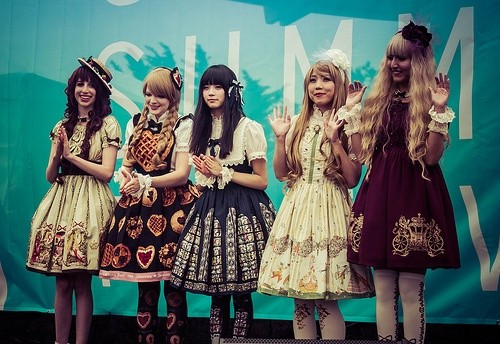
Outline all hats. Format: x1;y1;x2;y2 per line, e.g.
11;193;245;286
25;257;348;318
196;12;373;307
77;56;115;94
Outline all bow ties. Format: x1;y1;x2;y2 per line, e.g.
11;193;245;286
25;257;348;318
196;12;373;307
207;137;221;157
147;119;163;134
393;89;411;98
77;115;89;124
305;125;324;142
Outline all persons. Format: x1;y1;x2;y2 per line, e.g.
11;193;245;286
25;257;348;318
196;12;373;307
257;49;376;339
170;64;277;340
98;67;196;344
336;21;456;344
26;57;122;344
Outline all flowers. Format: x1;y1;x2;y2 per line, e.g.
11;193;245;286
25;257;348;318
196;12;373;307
401;20;433;47
328;48;349;69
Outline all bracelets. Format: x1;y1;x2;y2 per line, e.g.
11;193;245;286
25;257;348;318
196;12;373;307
331;138;342;144
